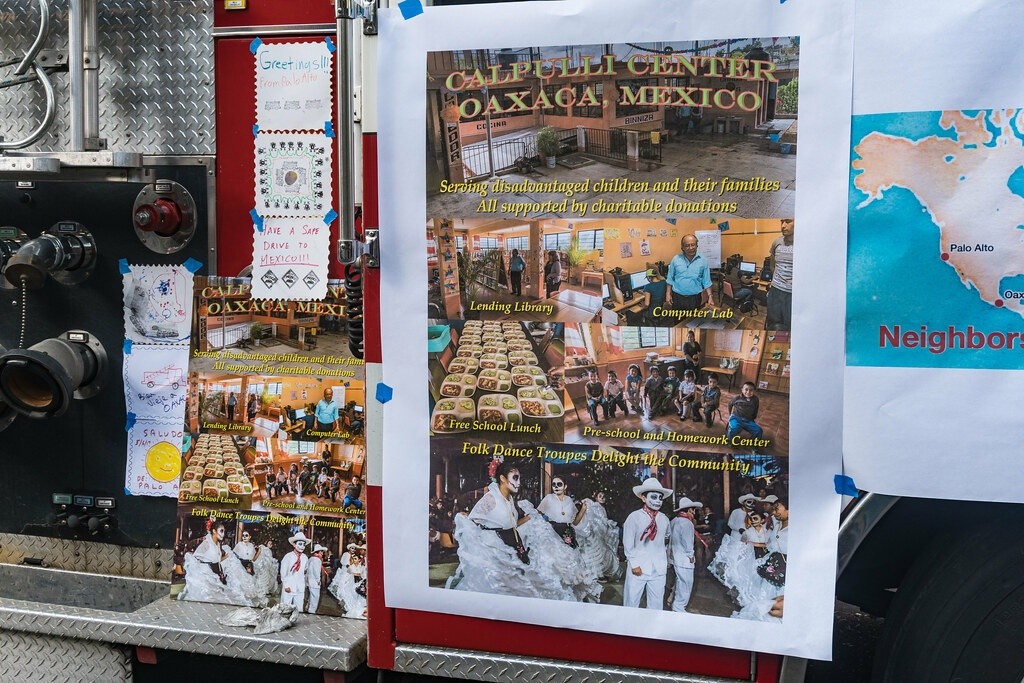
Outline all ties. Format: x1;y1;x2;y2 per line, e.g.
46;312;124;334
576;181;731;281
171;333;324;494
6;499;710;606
313;553;330;577
640;506;658;540
679;513;708;547
290;550;302;572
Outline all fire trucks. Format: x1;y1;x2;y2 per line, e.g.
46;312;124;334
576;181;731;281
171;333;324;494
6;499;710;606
0;0;1024;683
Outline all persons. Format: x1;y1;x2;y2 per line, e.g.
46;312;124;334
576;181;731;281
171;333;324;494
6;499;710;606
725;259;757;309
683;330;702;384
457;252;465;291
767;218;794;332
642;268;667;326
429;462;789;624
585;364;762;455
666;233;714;325
544;250;561;299
178;388;368;620
508;249;527;296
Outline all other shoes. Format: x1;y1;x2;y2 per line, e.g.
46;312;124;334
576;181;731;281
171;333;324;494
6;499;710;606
510;291;516;294
594;404;759;455
516;293;521;295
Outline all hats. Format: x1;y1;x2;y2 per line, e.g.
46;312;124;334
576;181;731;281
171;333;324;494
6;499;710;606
738;493;762;504
347;543;358;550
673;497;704;512
633;477;673;499
643;269;657;279
288;531;312;546
760;494;778;503
311;543;329;553
358;544;367;549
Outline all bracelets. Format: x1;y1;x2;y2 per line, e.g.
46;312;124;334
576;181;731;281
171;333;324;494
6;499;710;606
708;294;712;297
